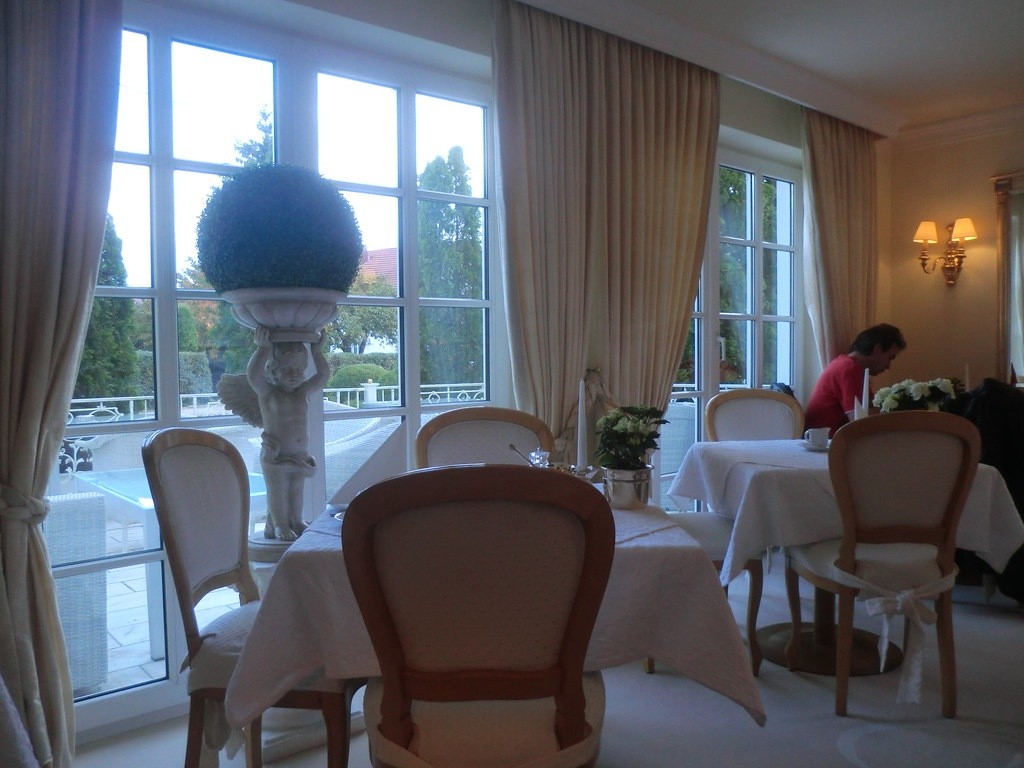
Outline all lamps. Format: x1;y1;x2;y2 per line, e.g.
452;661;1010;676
912;216;978;288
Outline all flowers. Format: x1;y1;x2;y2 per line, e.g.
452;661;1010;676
871;377;957;413
593;403;671;469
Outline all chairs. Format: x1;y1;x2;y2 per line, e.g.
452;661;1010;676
595;394;765;676
704;387;805;442
415;407;557;471
141;428;370;768
342;462;615;767
783;411;981;721
45;398;440;768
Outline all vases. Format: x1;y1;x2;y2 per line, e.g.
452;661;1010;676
602;465;653;510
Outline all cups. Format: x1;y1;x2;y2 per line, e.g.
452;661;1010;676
805;428;831;448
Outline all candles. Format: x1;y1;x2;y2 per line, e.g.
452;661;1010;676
861;368;871;412
577;377;588;467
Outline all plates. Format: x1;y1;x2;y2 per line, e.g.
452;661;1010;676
334;511;345;520
801;443;829;450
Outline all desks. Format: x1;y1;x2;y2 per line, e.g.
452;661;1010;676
666;438;1024;679
223;480;767;728
75;468;269;664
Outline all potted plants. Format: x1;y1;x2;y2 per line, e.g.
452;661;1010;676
197;161;363;344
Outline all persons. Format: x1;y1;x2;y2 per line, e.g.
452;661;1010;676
246;326;330;540
805;323;906;439
